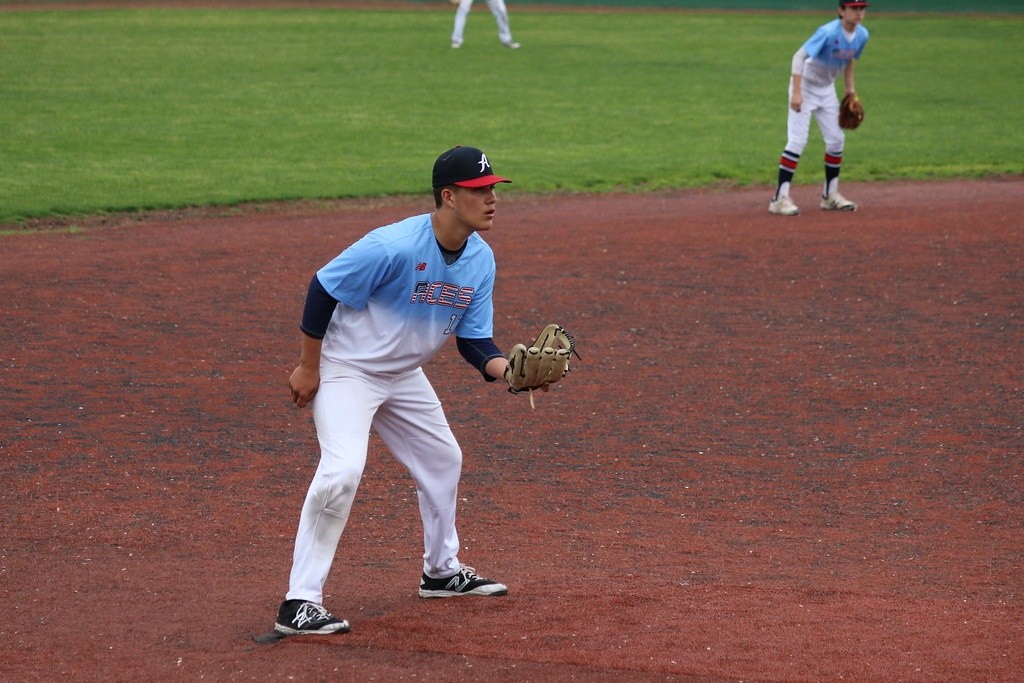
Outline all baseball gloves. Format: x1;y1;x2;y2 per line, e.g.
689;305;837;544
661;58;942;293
838;91;865;130
502;322;583;411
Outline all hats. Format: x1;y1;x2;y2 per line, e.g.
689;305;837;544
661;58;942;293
839;0;869;6
432;145;513;188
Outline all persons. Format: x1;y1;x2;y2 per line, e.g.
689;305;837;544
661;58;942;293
449;0;521;50
275;145;573;636
767;0;872;215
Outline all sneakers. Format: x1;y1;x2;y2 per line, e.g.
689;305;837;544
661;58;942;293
420;565;508;597
274;599;350;635
819;192;856;212
768;196;798;216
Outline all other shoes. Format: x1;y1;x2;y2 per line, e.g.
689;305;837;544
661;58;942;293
451;41;461;48
505;40;520;48
